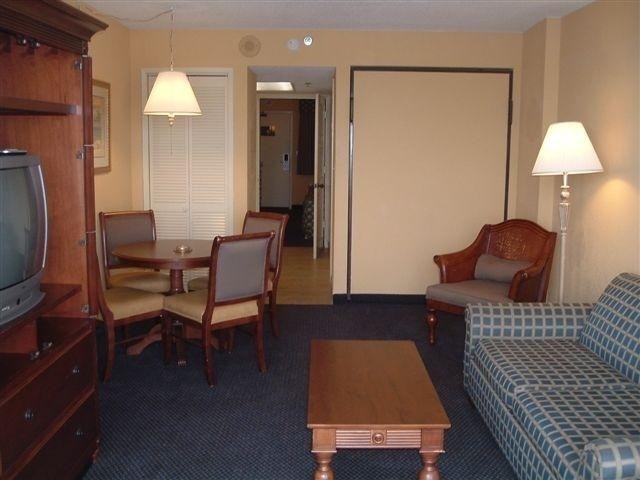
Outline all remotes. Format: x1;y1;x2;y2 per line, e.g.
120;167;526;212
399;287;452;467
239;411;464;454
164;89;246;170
0;149;28;155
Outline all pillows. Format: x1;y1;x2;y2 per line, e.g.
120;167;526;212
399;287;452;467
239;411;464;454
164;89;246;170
475;254;530;284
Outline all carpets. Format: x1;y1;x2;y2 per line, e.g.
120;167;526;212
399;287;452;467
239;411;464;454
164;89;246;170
85;304;518;477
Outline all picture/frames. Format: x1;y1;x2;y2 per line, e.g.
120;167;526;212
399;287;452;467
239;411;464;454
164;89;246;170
91;78;111;173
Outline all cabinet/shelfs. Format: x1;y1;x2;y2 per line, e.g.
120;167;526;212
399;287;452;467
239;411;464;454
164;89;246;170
0;0;102;480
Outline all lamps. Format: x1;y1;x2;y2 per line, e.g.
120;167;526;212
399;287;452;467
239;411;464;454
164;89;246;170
533;122;604;303
143;8;202;124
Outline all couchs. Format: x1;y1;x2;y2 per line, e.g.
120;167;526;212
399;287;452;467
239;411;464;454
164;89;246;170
462;271;638;480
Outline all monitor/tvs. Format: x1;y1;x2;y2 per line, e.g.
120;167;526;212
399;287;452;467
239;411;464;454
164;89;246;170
0;155;49;327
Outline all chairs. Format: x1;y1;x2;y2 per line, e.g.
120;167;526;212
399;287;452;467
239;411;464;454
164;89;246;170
425;217;558;345
97;269;170;380
188;210;288;346
167;231;276;388
97;210;171;294
